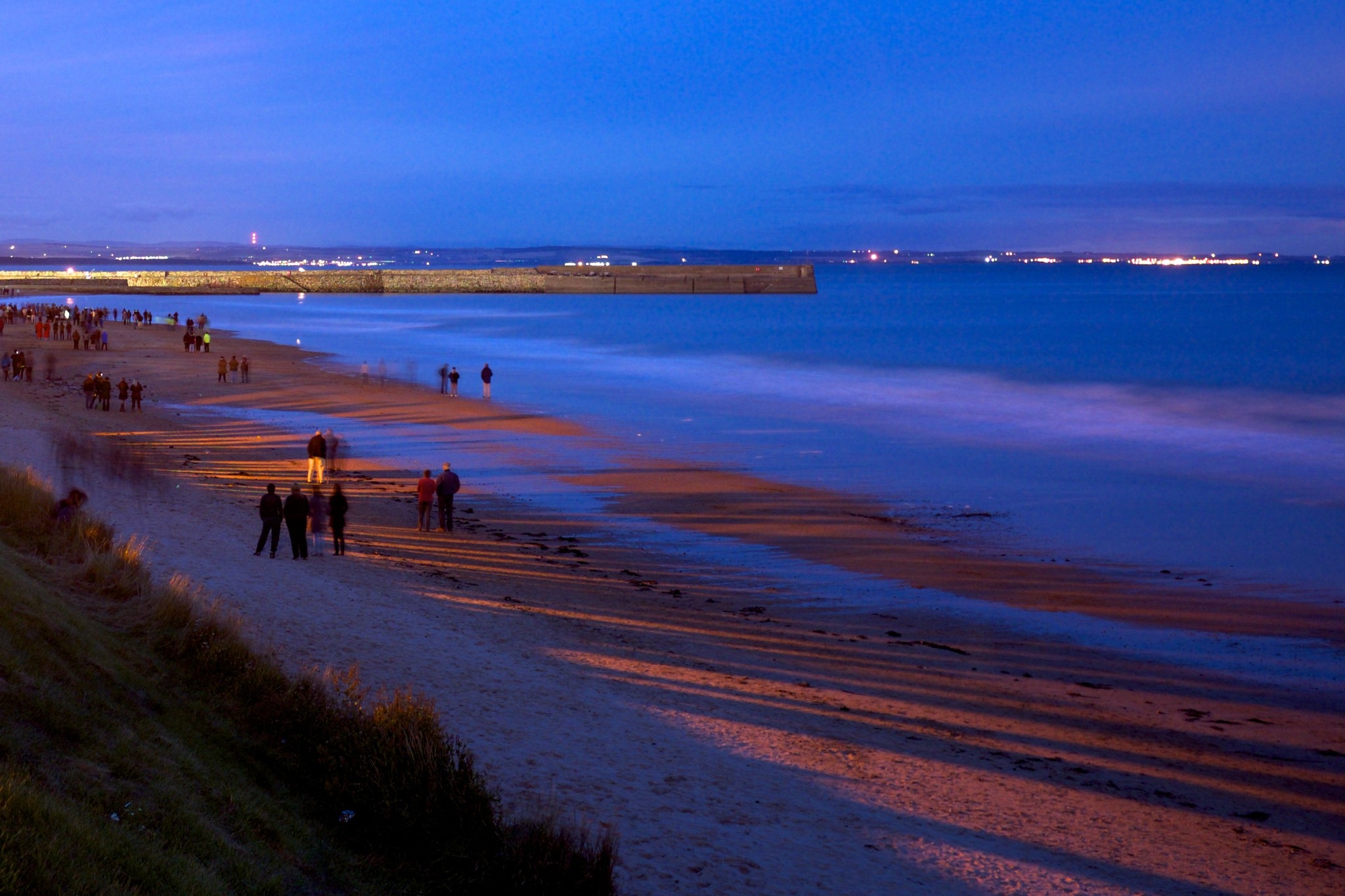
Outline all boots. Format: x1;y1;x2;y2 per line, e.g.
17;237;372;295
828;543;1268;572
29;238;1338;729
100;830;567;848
332;549;344;556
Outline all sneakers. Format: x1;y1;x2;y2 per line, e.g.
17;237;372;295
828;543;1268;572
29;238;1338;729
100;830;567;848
292;557;299;561
270;552;276;559
435;527;446;532
311;552;324;557
303;556;309;560
252;552;260;556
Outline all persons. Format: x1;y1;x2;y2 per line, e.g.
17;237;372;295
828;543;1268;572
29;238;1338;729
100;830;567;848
0;279;221;382
101;377;112;412
361;360;370;385
480;363;493;398
117;377;129;412
216;356;228;383
283;482;312;561
253;482;284;559
437;364;449;396
378;357;387;386
129;378;144;412
229;356;239;383
327;483;349;557
305;430;327;485
415;469;436;532
91;372;104;405
83;373;96;411
324;429;339;474
240;355;250;383
448;367;460;397
436;462;461;533
306;486;331;556
